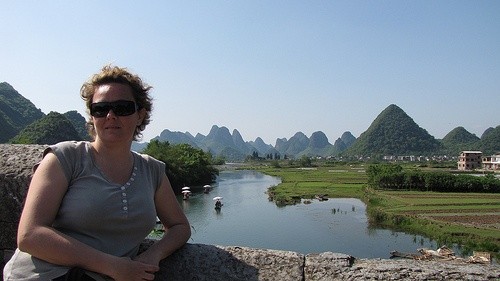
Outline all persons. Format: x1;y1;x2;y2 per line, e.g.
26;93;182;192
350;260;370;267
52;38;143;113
181;182;225;211
0;62;194;280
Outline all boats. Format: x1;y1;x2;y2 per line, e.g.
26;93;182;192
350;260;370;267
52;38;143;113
183;192;189;200
215;203;222;209
203;188;209;193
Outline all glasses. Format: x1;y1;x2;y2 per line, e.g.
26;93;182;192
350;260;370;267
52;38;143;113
89;100;141;119
